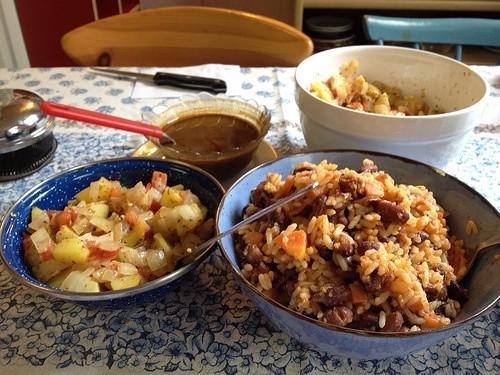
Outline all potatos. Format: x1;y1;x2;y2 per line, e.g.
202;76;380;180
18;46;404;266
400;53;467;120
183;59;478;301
310;60;443;116
23;171;214;293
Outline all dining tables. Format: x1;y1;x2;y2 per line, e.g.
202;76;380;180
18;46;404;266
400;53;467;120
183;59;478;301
0;64;500;375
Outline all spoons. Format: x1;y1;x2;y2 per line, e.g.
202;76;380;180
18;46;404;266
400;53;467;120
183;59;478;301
452;231;500;284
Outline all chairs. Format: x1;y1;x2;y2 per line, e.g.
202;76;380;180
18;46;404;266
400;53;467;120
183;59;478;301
60;6;314;67
363;14;500;66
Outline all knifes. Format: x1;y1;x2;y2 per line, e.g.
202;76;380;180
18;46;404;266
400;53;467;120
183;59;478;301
84;64;228;94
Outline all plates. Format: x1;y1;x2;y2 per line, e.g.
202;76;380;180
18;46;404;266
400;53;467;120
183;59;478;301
130;138;278;192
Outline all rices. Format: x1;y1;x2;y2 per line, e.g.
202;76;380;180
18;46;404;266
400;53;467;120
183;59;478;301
237;159;500;332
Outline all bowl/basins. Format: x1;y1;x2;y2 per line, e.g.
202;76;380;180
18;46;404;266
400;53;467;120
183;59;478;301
215;147;500;361
305;15;357;52
293;45;489;171
0;155;226;312
139;93;273;180
